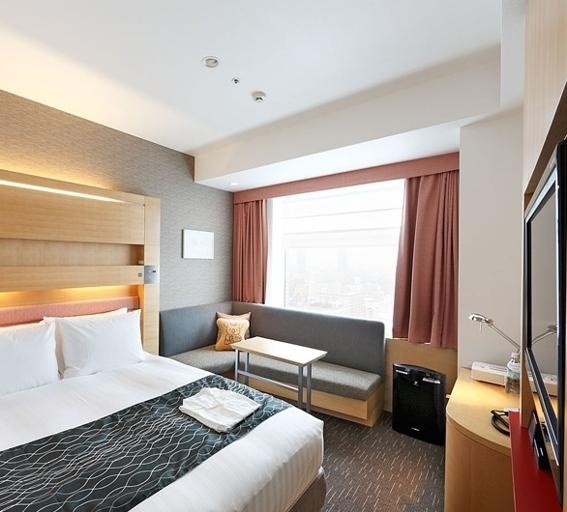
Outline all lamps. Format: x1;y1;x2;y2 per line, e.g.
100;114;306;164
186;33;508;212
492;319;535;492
531;325;557;346
468;313;519;361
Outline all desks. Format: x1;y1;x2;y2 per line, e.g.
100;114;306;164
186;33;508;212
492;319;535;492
230;336;328;415
444;367;519;512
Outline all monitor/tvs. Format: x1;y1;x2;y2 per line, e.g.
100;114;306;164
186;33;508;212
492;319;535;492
522;138;567;507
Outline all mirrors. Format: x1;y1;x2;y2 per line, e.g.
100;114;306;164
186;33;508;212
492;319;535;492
521;138;559;465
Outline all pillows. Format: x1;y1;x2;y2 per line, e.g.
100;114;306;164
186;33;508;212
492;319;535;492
215;312;252;350
0;306;146;395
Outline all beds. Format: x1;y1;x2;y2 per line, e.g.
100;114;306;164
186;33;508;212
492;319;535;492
0;296;326;512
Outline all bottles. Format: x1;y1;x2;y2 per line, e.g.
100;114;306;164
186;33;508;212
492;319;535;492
504;352;519;395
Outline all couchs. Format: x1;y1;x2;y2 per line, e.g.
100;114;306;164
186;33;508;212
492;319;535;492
159;299;386;428
